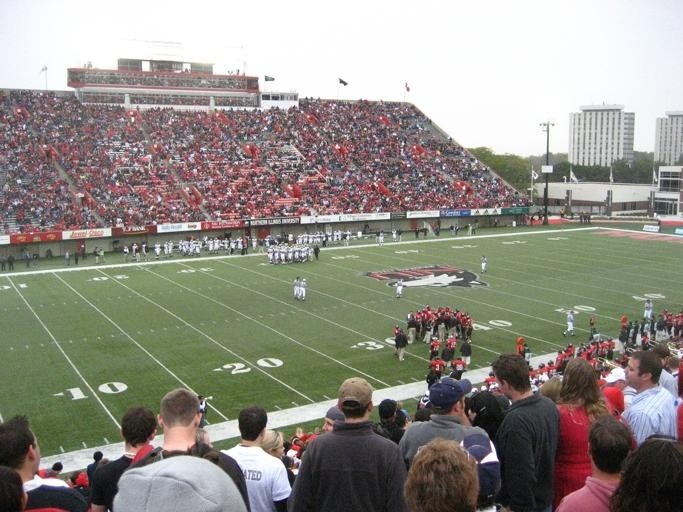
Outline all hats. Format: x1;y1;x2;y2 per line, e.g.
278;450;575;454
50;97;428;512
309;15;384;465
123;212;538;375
430;377;473;406
459;434;501;497
325;406;344;422
337;377;373;411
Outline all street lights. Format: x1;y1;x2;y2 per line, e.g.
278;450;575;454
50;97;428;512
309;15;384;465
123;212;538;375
537;119;557;225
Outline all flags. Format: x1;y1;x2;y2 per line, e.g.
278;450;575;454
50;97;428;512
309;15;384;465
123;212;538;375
651;169;657;183
608;166;614;185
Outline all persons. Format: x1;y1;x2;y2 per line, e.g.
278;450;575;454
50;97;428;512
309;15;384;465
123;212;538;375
479;255;488;274
395;278;403;298
292;275;299;299
297;277;307;302
